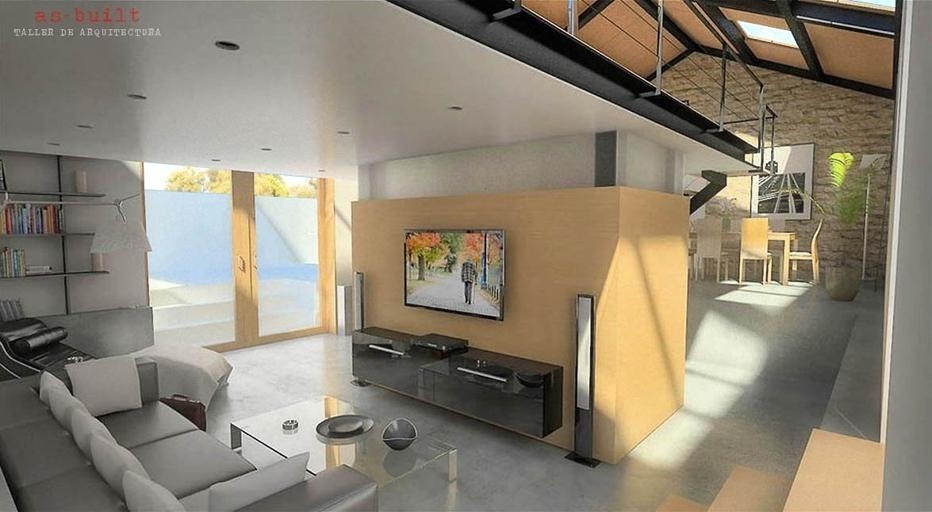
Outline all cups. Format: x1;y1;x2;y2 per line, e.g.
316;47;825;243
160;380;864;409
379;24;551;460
75;169;87;193
90;253;102;272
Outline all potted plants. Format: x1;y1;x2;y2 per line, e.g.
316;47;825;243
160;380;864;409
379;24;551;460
771;150;888;301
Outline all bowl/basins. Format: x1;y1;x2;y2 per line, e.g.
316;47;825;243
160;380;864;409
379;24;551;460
381;417;417;452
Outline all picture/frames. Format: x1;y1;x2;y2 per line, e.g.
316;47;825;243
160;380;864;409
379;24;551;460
750;142;815;221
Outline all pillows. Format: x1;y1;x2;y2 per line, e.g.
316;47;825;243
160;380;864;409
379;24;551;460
37;357;189;512
206;453;308;512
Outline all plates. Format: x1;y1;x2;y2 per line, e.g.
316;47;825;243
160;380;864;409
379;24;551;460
329;417;364;434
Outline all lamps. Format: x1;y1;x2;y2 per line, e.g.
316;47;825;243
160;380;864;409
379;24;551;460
0;192;152;255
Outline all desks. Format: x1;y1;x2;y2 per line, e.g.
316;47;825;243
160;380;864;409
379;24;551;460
689;232;797;286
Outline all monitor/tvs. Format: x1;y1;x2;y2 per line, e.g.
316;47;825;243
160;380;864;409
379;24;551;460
401;228;506;321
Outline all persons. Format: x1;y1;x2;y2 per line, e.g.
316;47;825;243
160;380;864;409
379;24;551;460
461;255;478;303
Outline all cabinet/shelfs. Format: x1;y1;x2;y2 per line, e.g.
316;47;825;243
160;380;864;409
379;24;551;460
0;155;110;314
351;326;563;438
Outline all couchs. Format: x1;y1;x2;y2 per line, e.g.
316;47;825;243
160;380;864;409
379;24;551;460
0;357;378;512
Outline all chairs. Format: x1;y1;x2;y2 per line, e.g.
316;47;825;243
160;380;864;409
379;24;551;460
693;216;729;283
779;218;824;286
0;319;232;406
739;218;772;287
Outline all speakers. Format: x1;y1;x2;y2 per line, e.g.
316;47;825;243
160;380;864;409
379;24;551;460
565;293;602;469
350;272;370;387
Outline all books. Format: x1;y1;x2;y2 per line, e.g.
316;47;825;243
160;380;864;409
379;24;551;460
0;297;25;323
0;203;63;236
0;245;53;278
